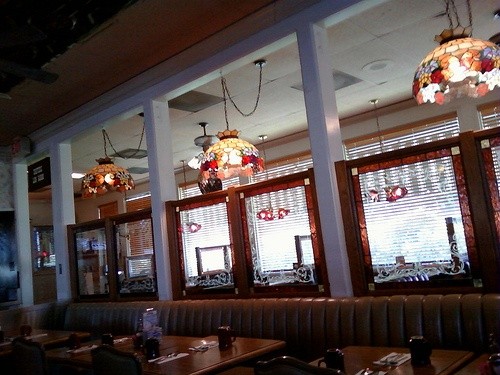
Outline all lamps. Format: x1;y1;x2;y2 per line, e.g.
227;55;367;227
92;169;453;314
194;122;223;193
366;99;408;202
257;135;289;220
81;128;135;200
201;79;264;179
180;160;202;233
412;0;500;105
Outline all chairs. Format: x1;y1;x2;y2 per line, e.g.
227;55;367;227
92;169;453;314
254;356;344;375
90;345;142;375
11;337;47;375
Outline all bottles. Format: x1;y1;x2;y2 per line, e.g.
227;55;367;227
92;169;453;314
133;313;144;355
488;333;499;372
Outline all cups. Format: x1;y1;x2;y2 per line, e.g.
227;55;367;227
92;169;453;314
408;335;433;366
145;338;160;360
69;331;81;350
217;326;236;351
101;332;114;346
0;330;5;344
19;325;32;337
317;348;345;372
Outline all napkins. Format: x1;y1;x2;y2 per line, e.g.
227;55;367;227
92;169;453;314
355;369;387;375
149;353;189;364
66;338;131;354
188;341;219;351
373;352;411;366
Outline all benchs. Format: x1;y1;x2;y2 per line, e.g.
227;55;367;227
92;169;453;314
66;293;500;354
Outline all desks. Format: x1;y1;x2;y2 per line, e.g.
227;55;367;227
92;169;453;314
455;354;492;375
141;335;285;375
0;327;90;356
47;335;201;365
309;345;474;375
